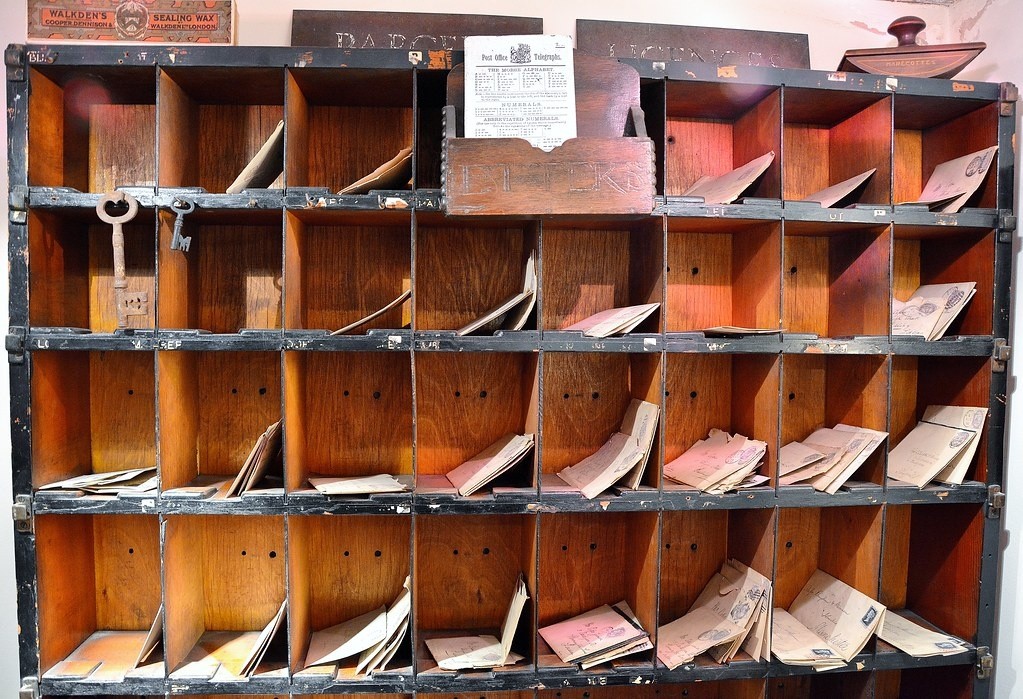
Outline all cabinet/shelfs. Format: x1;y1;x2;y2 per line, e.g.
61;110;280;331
5;43;1018;699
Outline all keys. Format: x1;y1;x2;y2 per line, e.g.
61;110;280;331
95;193;149;327
170;196;196;251
